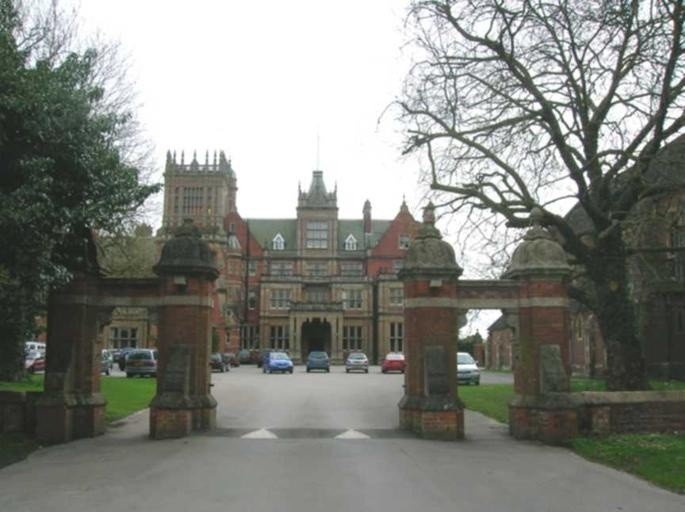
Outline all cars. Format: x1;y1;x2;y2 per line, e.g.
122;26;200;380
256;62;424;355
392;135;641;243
381;351;406;373
344;352;369;373
456;351;480;385
100;349;159;379
211;350;293;375
25;351;46;373
305;350;330;373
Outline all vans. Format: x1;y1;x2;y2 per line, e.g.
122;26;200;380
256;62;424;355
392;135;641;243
24;341;45;352
